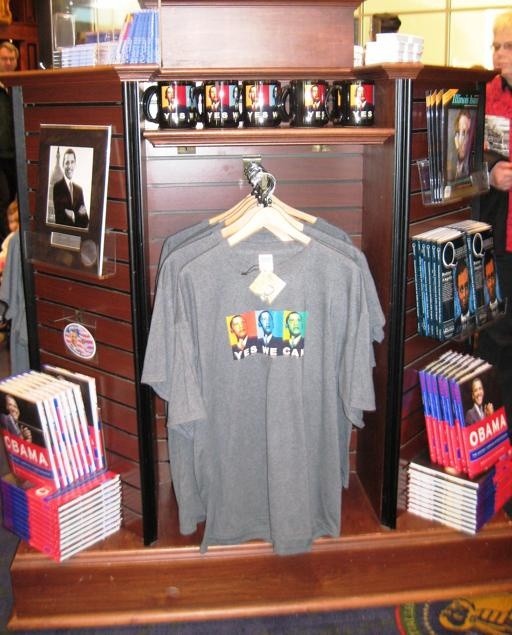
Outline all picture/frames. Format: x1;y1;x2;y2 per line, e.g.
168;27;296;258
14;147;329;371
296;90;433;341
26;121;113;279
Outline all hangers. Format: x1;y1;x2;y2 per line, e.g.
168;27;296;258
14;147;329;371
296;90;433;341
212;191;316;252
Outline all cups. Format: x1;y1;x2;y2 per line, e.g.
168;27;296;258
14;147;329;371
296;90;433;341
143;80;374;129
353;33;424;68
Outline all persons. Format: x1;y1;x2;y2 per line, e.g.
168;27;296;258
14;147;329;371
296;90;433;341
230;311;304;360
0;41;19;287
479;13;512;314
162;85;375;118
53;149;88;228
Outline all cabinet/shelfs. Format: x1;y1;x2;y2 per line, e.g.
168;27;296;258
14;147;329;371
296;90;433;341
0;0;511;632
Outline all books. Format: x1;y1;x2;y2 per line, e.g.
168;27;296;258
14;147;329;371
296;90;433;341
53;8;160;69
425;88;484;203
412;219;508;341
0;363;123;564
407;349;512;535
